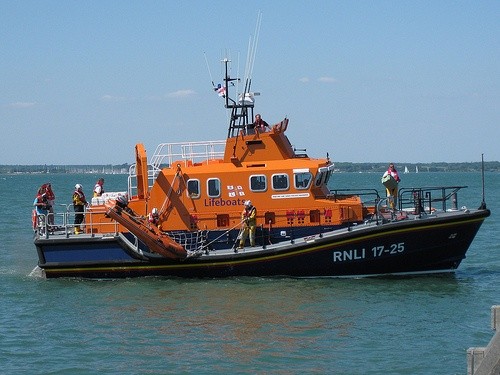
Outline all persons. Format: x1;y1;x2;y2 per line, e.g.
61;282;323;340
238;200;256;249
144;207;162;255
73;183;88;235
383;163;399;212
253;114;271;133
93;178;105;197
33;184;55;236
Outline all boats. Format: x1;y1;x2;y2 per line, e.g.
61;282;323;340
31;6;492;280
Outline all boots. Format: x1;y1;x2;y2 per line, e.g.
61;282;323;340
75;226;81;235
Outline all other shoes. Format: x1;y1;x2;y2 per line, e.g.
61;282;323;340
237;246;243;249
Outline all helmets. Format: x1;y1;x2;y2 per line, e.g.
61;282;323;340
75;184;82;189
244;201;251;208
152;208;158;215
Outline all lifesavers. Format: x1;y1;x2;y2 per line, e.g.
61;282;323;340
32;209;37;230
